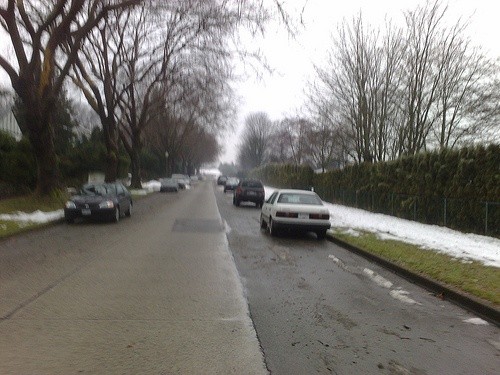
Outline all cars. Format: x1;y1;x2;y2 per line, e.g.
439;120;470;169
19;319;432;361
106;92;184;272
233;180;265;208
224;176;239;192
259;189;332;238
160;174;199;192
64;182;133;224
217;175;228;185
